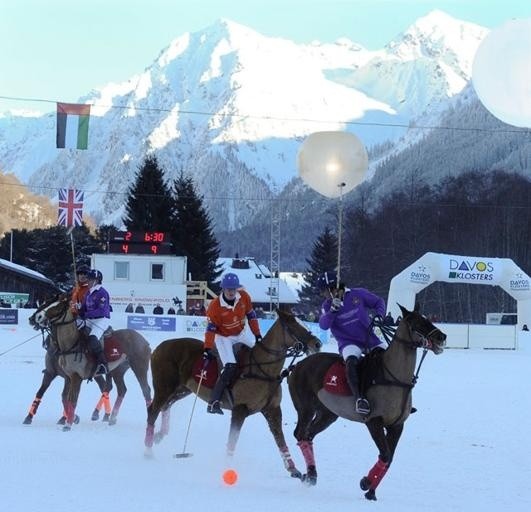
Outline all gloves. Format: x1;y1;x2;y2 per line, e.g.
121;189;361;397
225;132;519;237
373;316;382;326
204;350;213;360
330;299;343;311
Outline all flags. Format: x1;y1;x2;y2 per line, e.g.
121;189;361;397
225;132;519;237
57;102;91;149
58;189;84;227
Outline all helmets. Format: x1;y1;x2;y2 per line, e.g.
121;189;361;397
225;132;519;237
314;271;338;288
76;264;104;281
218;273;242;289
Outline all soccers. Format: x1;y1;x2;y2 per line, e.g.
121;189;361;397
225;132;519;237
223;470;237;484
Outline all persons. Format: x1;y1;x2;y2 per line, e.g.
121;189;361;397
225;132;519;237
70;264;111;375
153;303;186;315
316;270;386;411
125;303;144;313
255;306;267;318
385;312;402;326
200;273;262;414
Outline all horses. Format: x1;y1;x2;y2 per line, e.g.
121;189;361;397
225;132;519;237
23;289;152;433
141;305;323;479
280;301;447;501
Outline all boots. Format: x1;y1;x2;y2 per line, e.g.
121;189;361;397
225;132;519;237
94;349;109;374
345;367;368;410
207;378;226;415
